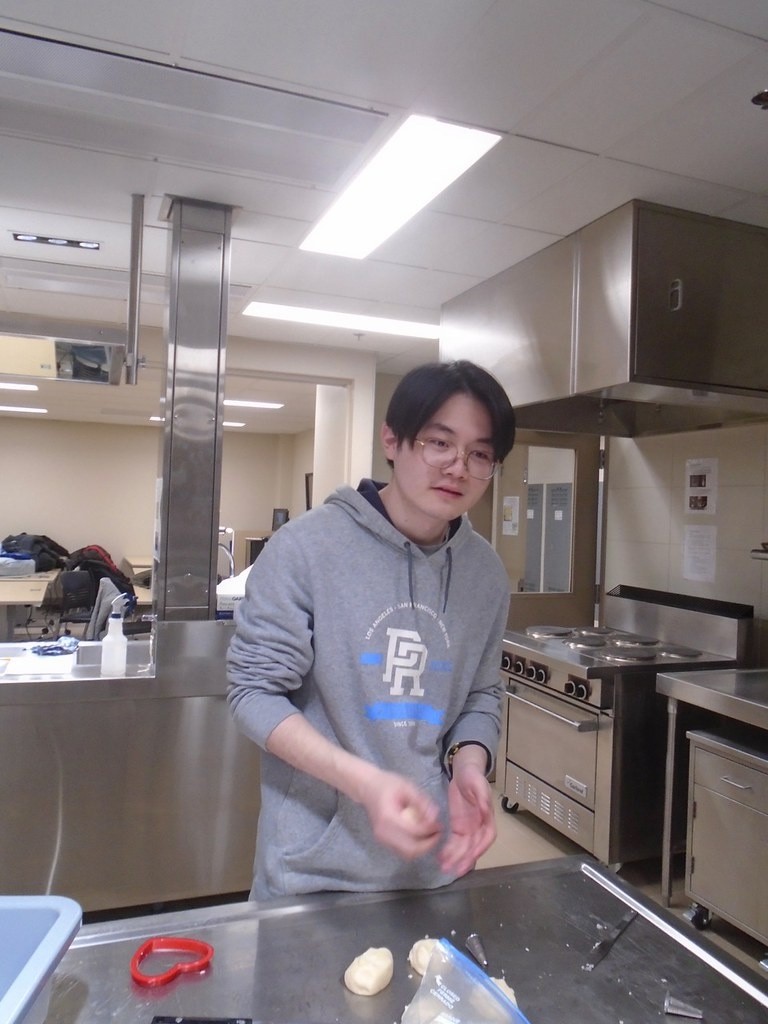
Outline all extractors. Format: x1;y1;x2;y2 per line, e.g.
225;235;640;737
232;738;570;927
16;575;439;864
434;203;768;436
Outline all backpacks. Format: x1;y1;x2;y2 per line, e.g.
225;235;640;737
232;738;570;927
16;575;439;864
42;545;136;619
3;532;70;572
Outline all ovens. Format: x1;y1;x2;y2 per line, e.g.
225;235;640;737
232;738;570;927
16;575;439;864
493;671;617;858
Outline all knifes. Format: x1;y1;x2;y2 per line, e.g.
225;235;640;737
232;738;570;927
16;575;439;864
583;911;637;972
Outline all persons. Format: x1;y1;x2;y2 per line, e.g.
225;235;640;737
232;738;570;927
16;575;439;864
225;360;517;897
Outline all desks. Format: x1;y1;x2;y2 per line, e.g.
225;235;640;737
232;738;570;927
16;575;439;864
32;851;768;1024
0;553;155;642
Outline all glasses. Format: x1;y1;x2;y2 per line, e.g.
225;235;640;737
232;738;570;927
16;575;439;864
415;438;500;480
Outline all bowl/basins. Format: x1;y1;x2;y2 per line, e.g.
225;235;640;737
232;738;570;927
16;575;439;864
0;894;83;1024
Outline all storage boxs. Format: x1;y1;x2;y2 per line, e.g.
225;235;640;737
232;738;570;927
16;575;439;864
0;895;83;1024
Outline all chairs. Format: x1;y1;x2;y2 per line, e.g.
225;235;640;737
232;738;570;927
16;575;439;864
58;570;96;624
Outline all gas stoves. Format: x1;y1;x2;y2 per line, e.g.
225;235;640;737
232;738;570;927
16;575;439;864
501;584;753;708
